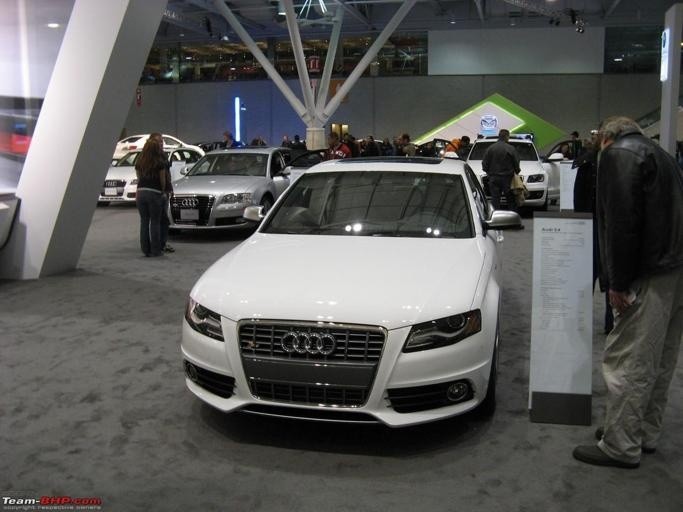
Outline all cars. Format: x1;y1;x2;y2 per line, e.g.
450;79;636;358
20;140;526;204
0;108;37;165
111;132;205;165
166;145;328;232
431;135;581;212
182;157;521;430
350;138;383;148
97;147;205;206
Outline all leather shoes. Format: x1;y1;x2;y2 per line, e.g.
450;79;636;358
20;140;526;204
572;441;642;470
595;425;658;455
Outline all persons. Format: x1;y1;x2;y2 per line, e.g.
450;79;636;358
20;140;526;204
135;138;166;257
548;116;683;470
423;143;435;157
447;136;471;153
220;132;240;149
481;130;521;211
323;131;421;160
291;135;307;150
251;136;267;146
281;136;292;147
149;133;173;252
